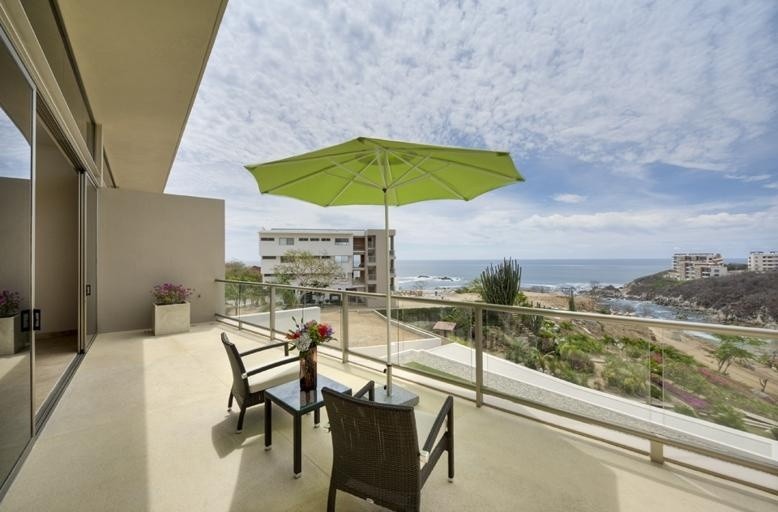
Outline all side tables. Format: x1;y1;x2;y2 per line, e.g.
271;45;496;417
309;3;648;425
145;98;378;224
263;375;351;479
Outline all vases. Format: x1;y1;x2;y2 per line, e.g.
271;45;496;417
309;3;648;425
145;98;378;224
152;301;191;336
299;344;318;391
1;317;25;355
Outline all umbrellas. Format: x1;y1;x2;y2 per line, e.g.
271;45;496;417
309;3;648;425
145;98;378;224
242;136;526;398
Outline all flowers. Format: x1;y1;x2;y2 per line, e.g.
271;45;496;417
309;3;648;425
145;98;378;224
0;287;23;316
151;283;192;303
288;320;337;352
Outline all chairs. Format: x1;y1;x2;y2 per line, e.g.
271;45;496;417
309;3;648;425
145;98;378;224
321;380;455;512
220;332;299;434
